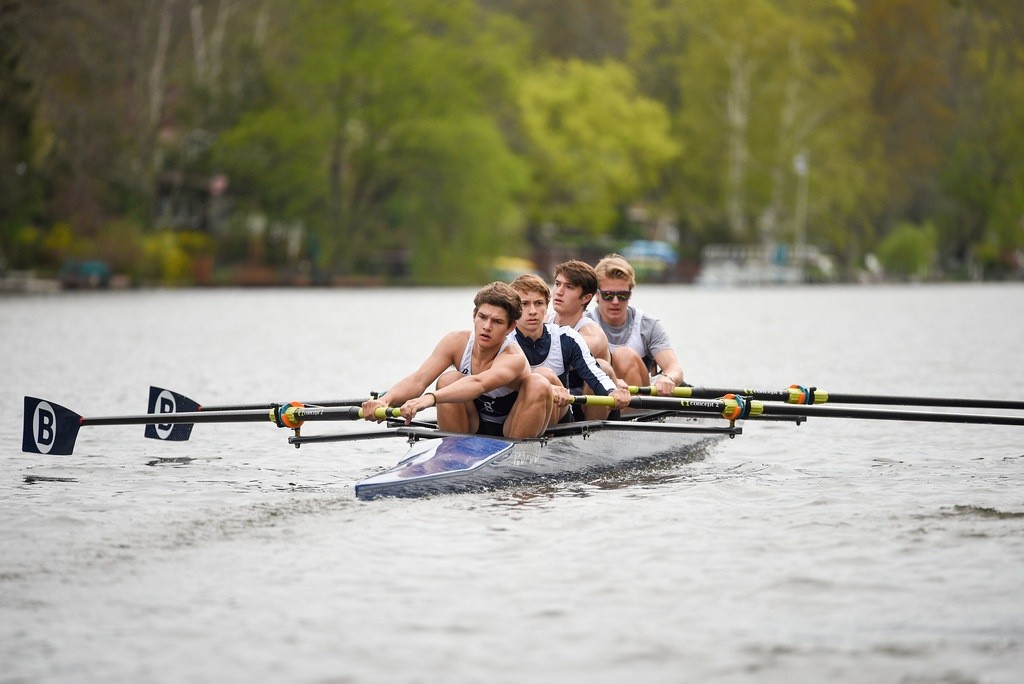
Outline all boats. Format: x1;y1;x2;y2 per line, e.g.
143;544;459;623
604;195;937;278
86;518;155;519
352;409;764;499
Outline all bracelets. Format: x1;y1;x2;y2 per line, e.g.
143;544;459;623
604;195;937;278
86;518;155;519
425;393;436;407
664;374;674;382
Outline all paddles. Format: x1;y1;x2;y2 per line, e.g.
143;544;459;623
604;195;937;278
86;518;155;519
144;385;371;442
20;395;402;456
553;393;1024;428
627;385;1024;410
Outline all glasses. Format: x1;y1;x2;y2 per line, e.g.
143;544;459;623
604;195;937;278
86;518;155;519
597;289;631;301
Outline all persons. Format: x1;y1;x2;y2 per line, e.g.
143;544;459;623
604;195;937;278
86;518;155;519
585;258;683;414
544;262;621;421
362;280;553;440
509;274;631;427
